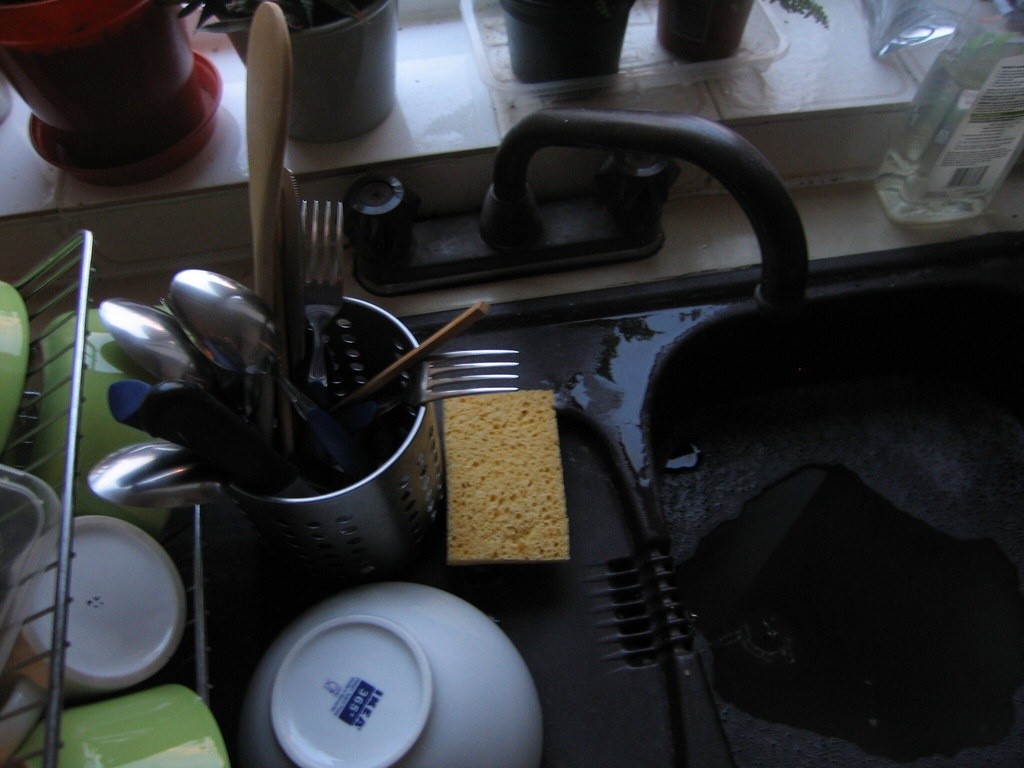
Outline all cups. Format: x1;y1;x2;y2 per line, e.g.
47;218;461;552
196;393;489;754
6;683;231;768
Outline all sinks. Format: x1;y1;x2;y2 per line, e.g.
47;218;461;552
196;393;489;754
628;226;1024;768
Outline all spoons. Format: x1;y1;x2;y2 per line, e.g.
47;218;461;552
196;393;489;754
100;296;215;393
88;438;225;509
163;267;361;477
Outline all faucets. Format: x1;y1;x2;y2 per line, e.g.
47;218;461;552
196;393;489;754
477;107;808;316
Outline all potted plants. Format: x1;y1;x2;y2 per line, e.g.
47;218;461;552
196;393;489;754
171;0;400;147
657;0;830;64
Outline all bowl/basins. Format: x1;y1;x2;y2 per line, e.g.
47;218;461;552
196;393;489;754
232;582;543;768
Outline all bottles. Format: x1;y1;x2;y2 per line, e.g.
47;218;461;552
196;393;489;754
875;1;1024;226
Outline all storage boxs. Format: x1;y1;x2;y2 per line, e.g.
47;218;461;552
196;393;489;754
459;0;792;118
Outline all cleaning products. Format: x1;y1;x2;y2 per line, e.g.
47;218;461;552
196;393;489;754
874;0;1024;226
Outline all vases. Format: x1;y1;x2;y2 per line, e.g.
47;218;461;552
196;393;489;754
499;0;638;97
0;0;206;168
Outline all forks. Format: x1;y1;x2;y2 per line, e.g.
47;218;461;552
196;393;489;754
292;198;346;393
408;345;523;407
